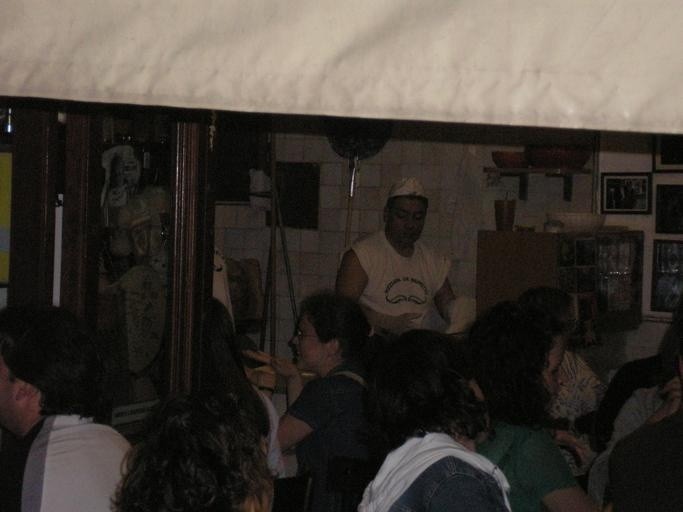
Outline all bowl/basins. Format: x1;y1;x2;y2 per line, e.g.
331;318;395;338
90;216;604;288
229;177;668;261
490;149;528;169
525;144;593;170
545;211;607;235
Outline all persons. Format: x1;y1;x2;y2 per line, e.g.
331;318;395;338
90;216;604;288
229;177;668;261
461;298;615;511
605;337;682;511
353;329;515;511
334;174;463;345
593;289;683;450
200;293;289;481
105;380;276;512
585;316;683;510
235;292;398;512
0;302;136;512
517;286;608;496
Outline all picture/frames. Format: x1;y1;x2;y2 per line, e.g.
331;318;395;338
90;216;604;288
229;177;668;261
652;134;683;174
651;240;683;312
600;171;654;215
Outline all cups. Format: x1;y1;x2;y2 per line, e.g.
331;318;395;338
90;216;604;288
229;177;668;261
494;199;515;231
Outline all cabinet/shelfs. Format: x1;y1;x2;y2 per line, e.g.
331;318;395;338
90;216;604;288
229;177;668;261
477;225;629;326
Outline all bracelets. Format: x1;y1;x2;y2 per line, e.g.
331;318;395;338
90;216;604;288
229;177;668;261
257;385;275;395
552;427;559;439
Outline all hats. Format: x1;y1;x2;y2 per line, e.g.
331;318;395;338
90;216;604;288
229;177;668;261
386;163;430;201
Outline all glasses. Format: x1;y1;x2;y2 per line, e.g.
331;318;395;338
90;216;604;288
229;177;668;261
297;330;317;341
